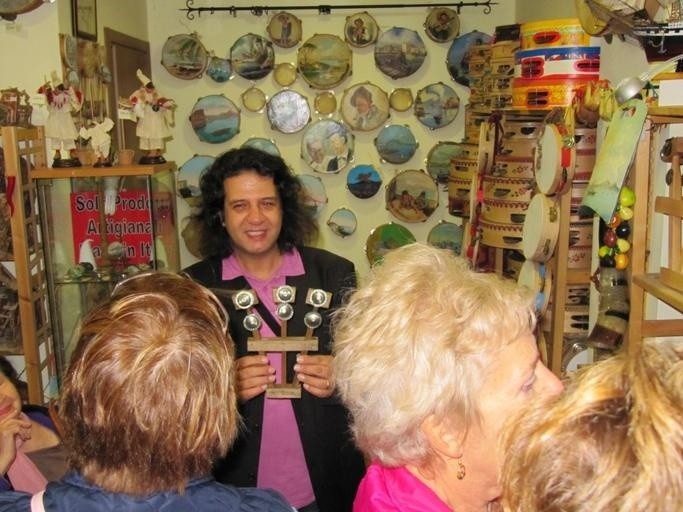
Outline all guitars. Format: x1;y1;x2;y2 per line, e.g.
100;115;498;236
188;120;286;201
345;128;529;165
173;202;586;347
573;1;677;48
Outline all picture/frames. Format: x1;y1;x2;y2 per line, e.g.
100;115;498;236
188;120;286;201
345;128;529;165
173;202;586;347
70;0;99;42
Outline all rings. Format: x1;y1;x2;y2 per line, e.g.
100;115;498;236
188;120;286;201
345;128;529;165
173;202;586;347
326;379;330;389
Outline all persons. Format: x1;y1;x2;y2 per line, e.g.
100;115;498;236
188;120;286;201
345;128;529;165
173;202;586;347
352;17;370;45
325;123;353;171
0;269;300;512
0;354;69;494
429;12;453;39
37;80;84;159
306;137;336;171
400;190;419;214
278;13;292;45
496;338;683;512
129;81;172;156
177;146;359;512
415;190;429;210
350;86;388;129
326;241;567;512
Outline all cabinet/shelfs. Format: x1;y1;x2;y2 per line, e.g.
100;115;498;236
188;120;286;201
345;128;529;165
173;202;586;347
627;107;683;358
1;123;184;409
